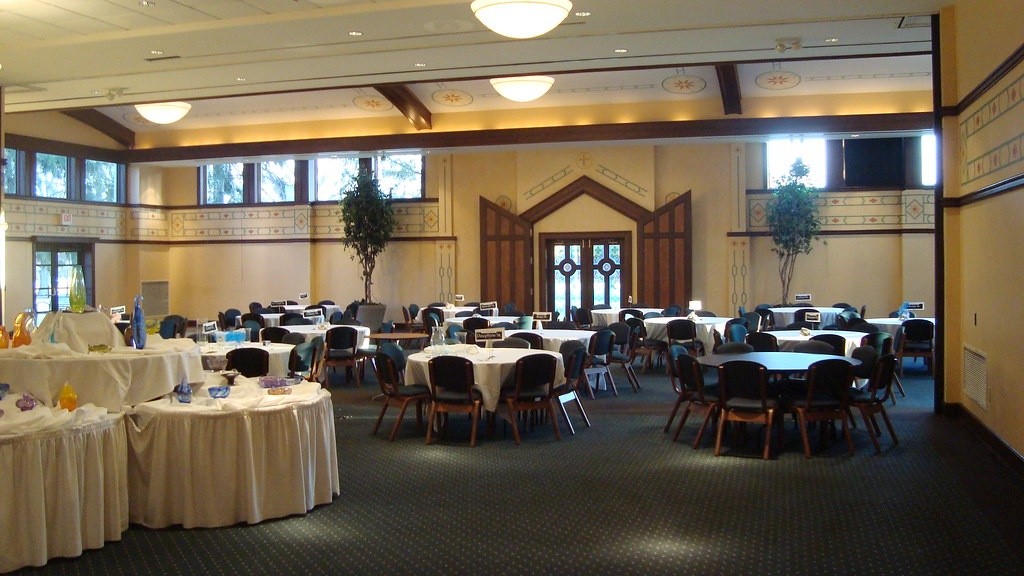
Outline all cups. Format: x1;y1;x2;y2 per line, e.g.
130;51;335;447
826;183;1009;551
454;332;467;346
196;318;209;355
430;327;446;356
242;328;252;343
0;383;10;401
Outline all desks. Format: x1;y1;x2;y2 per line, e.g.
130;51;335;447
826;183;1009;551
696;352;862;456
259;325;371;382
404;348;567;440
0;333;207;413
743;331;870;357
0;408;131;574
443;316;543;338
124;377;341;530
197;341;302;379
592;309;665;328
416;306;479;326
234;313;285;329
864;317;936;355
767;306;846;331
363;332;429;350
505;330;607;391
644;316;735;355
270;305;340;322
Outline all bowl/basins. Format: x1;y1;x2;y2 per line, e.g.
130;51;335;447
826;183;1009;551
209;386;230;398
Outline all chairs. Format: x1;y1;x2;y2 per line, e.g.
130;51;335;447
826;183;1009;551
112;300;936;462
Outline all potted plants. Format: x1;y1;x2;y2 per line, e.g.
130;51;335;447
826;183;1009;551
337;166;393;345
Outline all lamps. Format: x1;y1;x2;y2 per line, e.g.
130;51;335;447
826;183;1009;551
490;76;555;104
134;101;192;125
688;301;702;323
470;0;573;40
